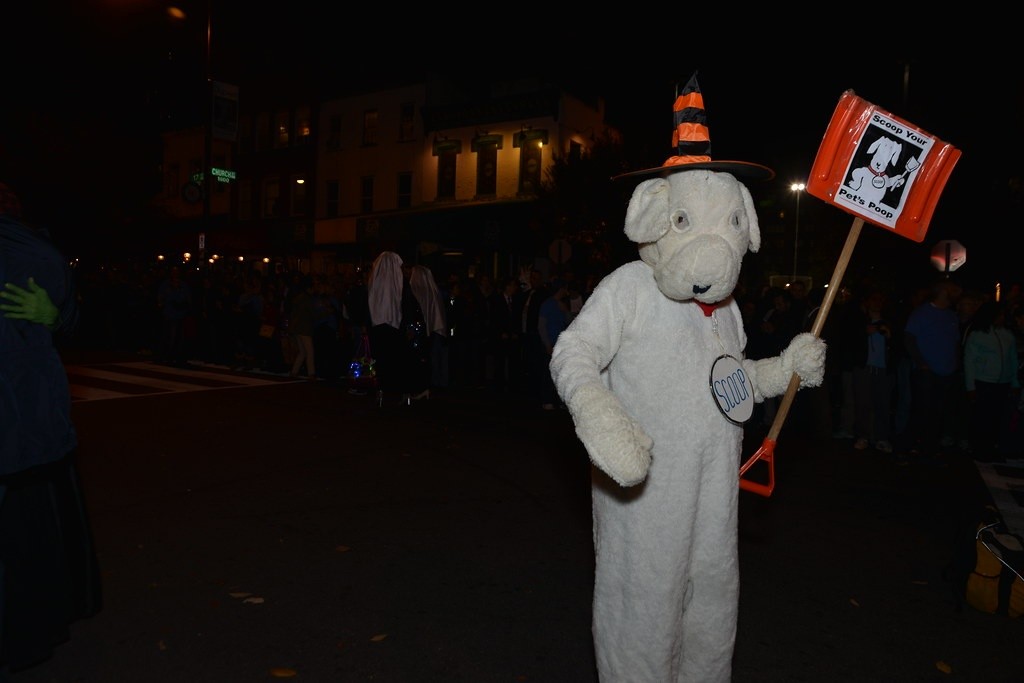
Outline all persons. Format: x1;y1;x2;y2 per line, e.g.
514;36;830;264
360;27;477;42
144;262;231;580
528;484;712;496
0;178;104;674
729;265;1024;458
83;248;608;402
1;272;86;335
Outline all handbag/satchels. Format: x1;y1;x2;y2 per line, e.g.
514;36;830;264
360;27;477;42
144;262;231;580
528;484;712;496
258;320;276;338
405;321;434;353
345;333;384;407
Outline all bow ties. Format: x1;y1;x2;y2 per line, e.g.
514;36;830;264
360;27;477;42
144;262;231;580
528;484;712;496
449;298;456;301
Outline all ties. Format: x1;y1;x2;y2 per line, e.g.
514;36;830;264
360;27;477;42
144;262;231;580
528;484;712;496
507;297;511;303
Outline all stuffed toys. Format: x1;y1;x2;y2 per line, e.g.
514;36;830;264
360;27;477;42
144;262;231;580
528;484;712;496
549;73;832;683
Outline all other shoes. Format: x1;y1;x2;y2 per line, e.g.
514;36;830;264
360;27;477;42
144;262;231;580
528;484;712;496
543;403;554;410
891;458;914;466
853;437;869;449
875;440;894;453
832;429;854;439
307;376;318;384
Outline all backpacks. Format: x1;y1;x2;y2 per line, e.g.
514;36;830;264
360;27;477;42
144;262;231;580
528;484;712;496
965;504;1024;619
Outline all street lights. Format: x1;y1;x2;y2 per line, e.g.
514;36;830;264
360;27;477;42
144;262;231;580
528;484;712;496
169;1;214;275
